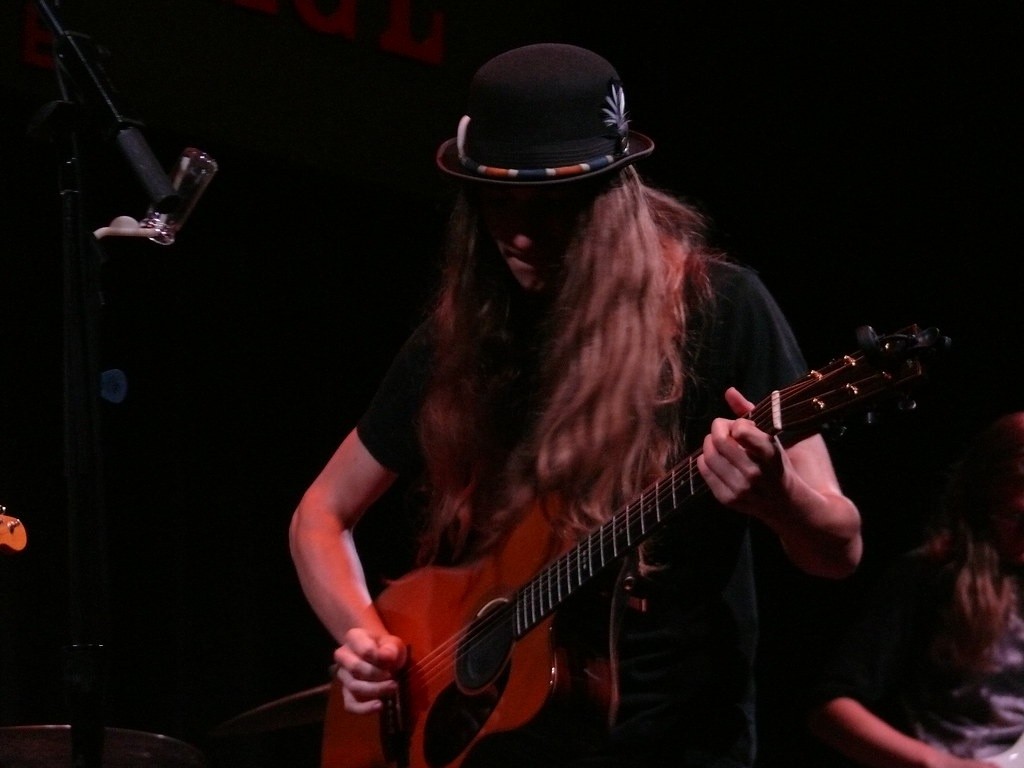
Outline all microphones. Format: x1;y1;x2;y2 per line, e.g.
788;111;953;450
146;147;217;245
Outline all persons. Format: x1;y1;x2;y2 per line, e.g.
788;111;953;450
813;409;1024;768
287;42;863;768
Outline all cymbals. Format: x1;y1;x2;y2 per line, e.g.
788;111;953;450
0;724;198;767
220;680;331;732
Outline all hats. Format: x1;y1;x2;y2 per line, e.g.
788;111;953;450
436;43;655;186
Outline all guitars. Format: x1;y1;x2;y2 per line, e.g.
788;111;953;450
301;311;957;768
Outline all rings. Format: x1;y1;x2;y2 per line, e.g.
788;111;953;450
330;661;343;677
